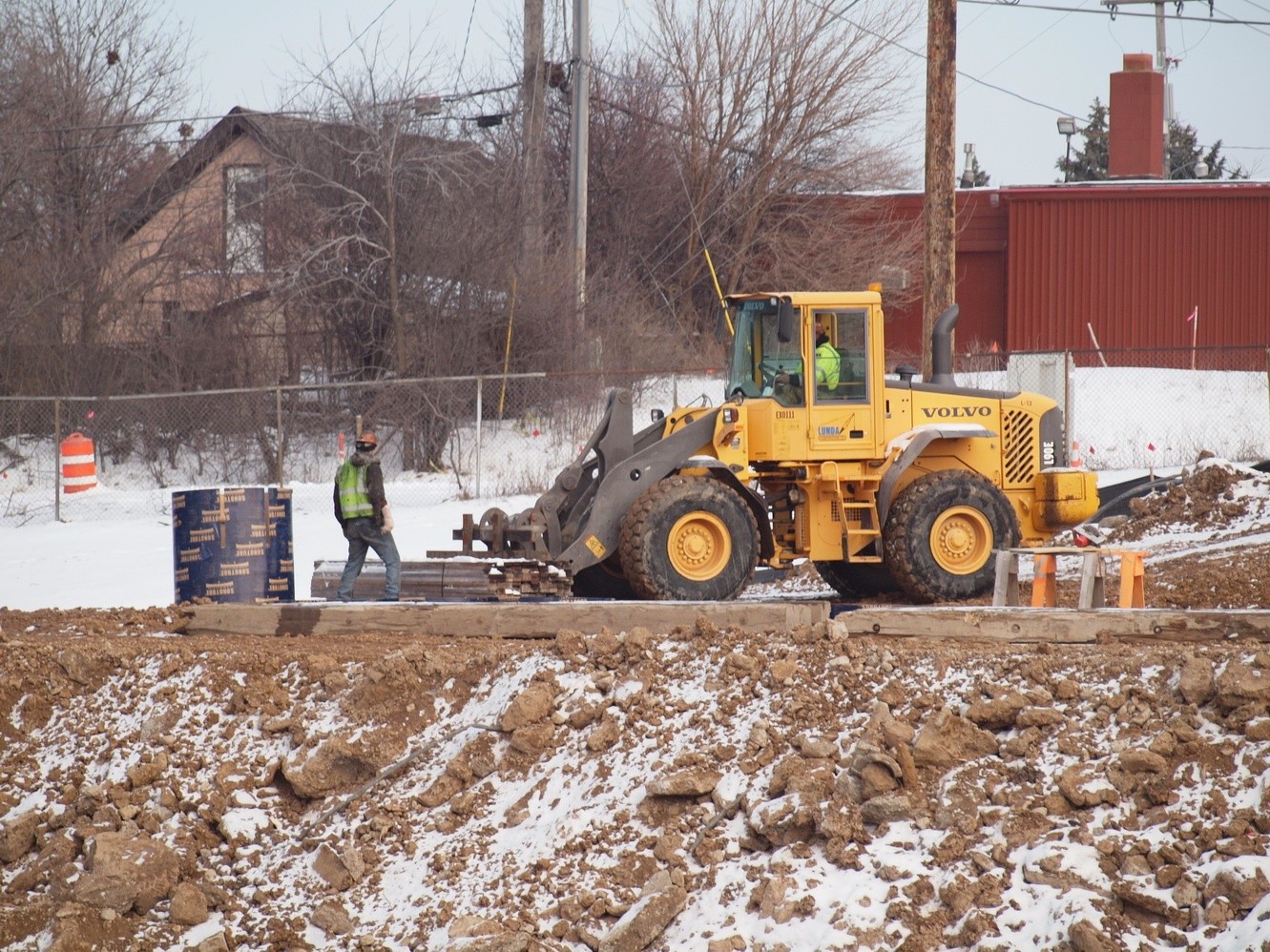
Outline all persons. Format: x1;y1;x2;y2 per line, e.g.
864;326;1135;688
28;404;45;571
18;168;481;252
774;320;841;407
333;430;403;602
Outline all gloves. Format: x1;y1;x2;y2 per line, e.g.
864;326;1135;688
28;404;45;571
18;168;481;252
380;505;393;535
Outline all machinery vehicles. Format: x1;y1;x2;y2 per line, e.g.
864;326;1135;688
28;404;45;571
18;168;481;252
310;292;1099;605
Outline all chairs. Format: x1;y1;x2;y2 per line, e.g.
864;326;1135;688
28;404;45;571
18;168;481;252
835;348;853;395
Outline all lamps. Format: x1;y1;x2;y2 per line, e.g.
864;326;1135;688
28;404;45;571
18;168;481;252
1057;117;1077;183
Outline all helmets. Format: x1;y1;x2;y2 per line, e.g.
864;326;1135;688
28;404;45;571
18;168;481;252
814;317;824;335
355;432;377;446
